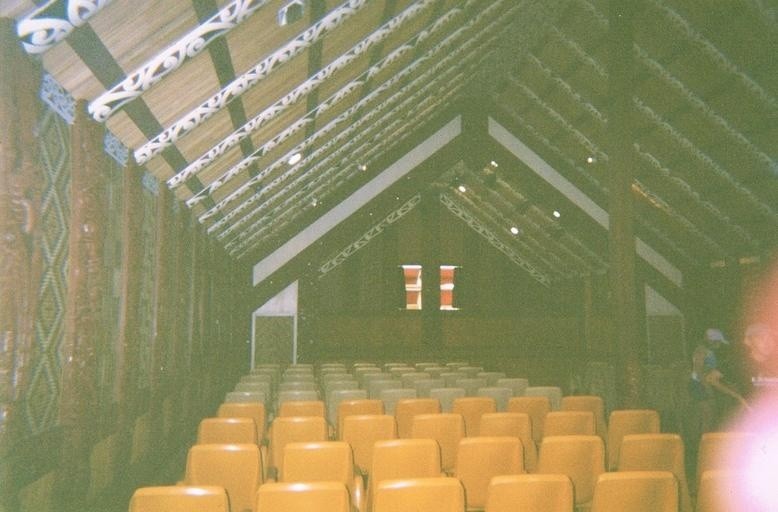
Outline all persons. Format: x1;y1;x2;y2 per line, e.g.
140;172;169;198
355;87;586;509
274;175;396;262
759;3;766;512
689;328;730;444
705;323;778;432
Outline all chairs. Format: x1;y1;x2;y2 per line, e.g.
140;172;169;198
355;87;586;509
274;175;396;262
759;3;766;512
125;353;761;511
